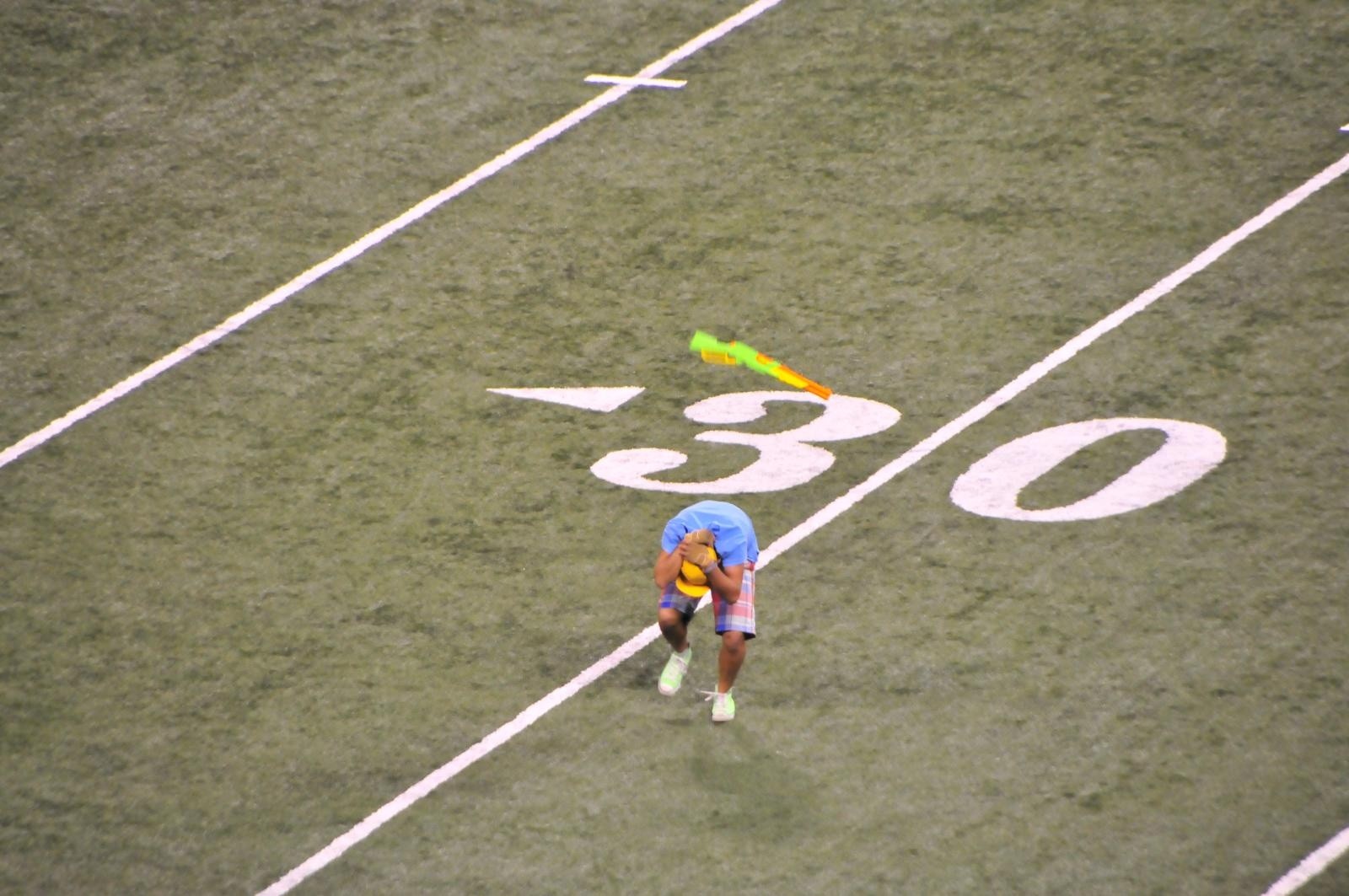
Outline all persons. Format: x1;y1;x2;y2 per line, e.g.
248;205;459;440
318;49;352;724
654;500;760;722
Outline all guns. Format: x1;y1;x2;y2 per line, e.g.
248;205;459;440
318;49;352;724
690;329;831;400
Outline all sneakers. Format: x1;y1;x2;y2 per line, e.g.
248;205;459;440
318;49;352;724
700;683;734;722
658;644;693;696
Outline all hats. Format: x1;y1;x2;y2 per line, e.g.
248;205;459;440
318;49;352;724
675;546;718;598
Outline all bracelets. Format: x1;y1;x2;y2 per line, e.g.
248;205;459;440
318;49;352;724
704;561;718;574
